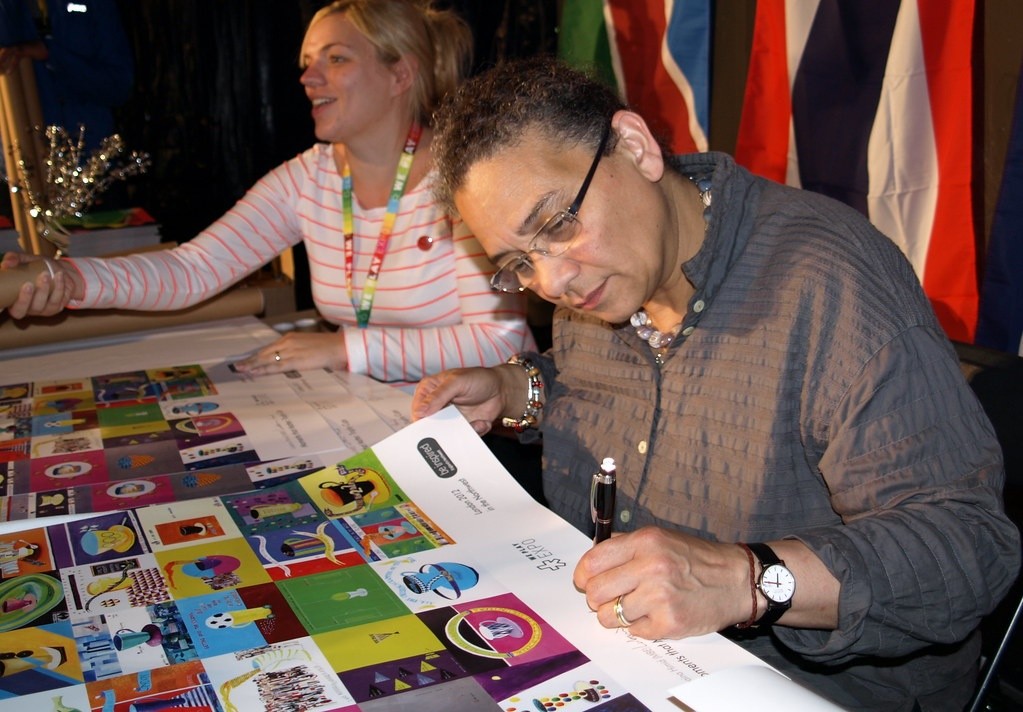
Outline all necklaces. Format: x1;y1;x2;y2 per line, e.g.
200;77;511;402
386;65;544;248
627;170;714;351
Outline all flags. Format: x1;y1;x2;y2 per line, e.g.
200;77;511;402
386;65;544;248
554;0;1022;352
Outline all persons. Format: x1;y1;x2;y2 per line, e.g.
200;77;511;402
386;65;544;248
406;58;1023;711
2;1;542;384
0;256;77;314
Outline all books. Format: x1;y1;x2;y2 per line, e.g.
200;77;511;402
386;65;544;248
54;206;162;255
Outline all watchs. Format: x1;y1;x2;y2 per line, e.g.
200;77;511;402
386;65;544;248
746;542;797;641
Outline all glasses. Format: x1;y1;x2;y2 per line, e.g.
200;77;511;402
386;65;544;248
488;102;629;293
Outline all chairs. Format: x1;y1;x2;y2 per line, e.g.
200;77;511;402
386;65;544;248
962;352;1023;712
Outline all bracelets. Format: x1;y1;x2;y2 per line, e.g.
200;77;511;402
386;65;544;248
733;541;758;629
500;353;543;429
43;257;55;280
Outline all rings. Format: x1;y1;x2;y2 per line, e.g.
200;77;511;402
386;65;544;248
614;596;633;628
275;351;281;361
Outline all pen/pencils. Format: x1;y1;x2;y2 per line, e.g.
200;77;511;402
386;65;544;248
589;455;616;543
